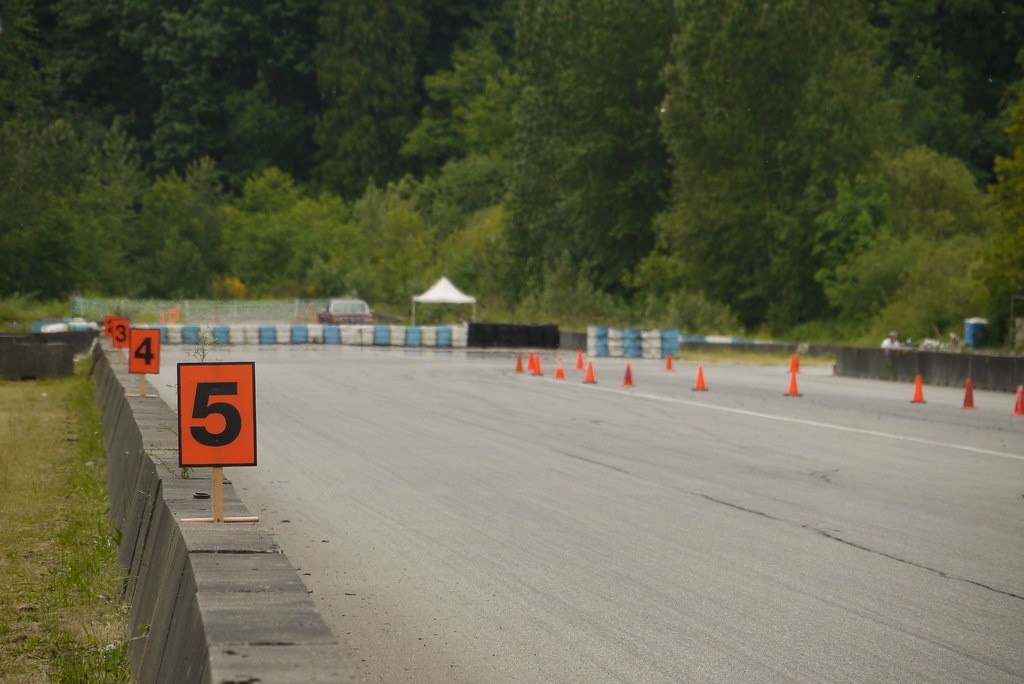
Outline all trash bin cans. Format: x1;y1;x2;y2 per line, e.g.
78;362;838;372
965;317;989;349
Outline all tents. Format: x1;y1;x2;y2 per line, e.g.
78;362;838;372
410;276;477;326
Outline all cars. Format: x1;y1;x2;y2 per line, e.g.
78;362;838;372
317;298;374;325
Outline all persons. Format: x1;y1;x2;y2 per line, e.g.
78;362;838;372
881;331;902;350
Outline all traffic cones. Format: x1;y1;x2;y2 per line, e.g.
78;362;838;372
515;355;523;372
575;349;584;370
787;354;801;374
910;374;926;404
527;352;535;371
664;353;674;371
1011;385;1024;417
531;356;543;376
582;362;596;384
555;367;565;379
624;363;632;386
691;365;709;392
784;372;803;397
959;378;977;410
